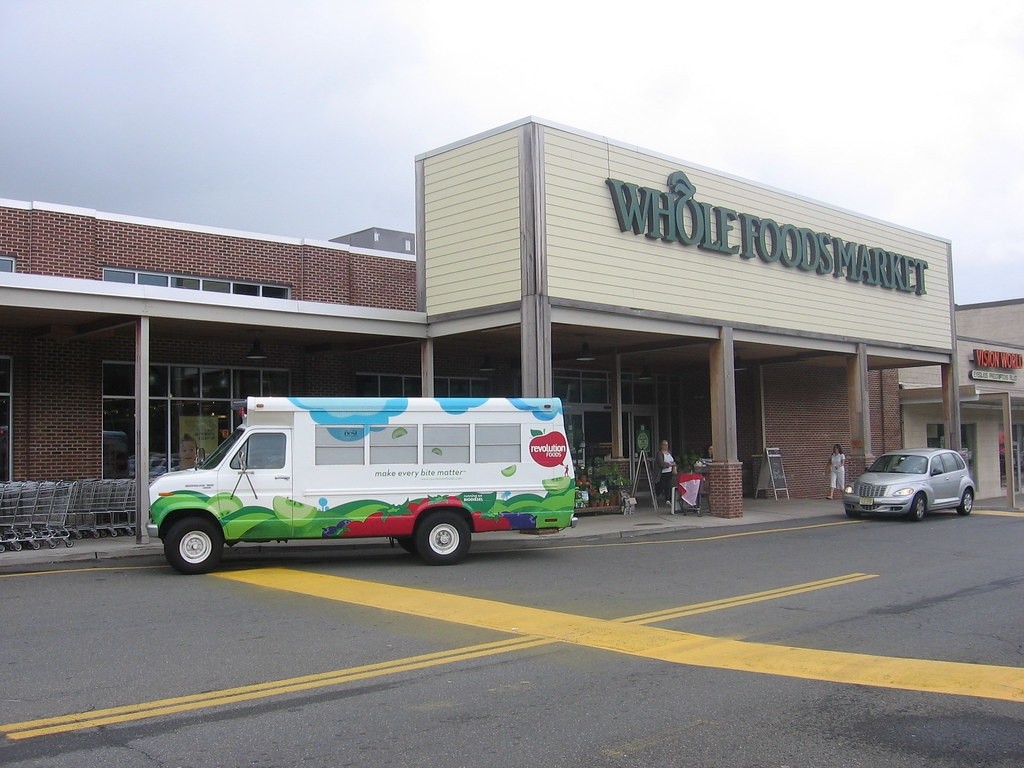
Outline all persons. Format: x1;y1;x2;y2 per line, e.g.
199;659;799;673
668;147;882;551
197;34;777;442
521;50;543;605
708;446;713;459
180;434;197;468
655;439;676;506
826;444;845;500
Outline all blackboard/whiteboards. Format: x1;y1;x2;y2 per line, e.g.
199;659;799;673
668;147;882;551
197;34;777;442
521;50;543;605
767;454;788;490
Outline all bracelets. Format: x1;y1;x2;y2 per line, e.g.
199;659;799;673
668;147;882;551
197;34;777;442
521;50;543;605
840;465;842;467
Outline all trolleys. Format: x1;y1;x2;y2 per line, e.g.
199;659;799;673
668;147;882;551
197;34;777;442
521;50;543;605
0;477;141;554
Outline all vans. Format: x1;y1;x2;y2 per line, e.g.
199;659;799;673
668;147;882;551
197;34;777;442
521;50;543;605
147;396;578;566
102;430;130;478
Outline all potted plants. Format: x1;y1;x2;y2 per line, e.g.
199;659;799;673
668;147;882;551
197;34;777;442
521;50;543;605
574;457;631;508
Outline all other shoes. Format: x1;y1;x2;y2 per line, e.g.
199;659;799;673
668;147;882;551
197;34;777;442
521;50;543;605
665;501;671;507
826;496;833;500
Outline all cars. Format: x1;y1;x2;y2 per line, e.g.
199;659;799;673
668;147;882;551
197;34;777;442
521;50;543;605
840;446;976;523
